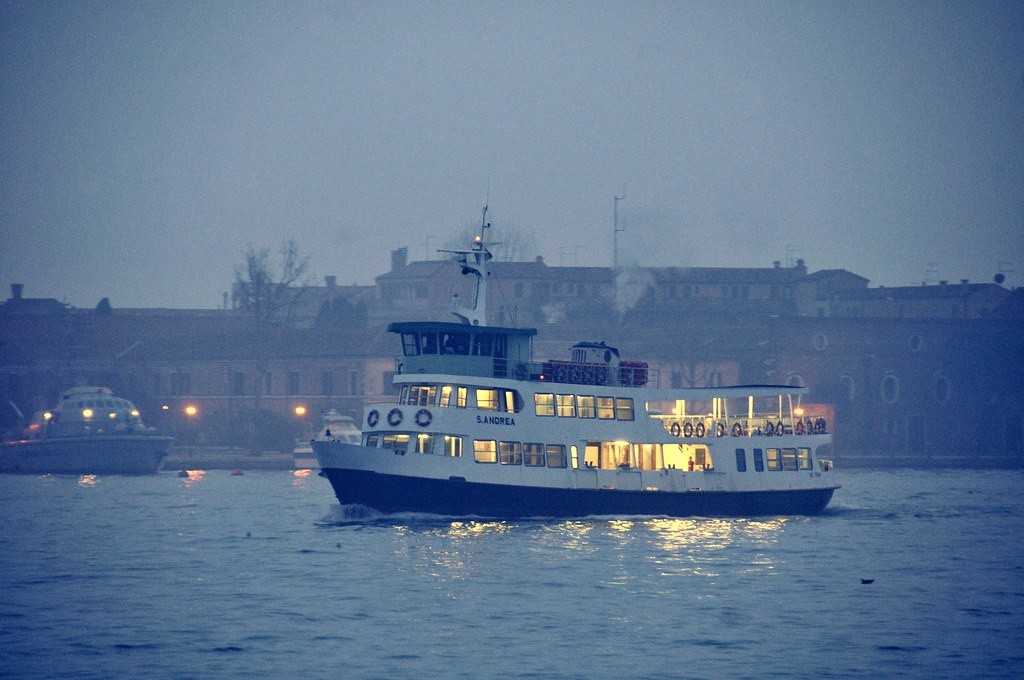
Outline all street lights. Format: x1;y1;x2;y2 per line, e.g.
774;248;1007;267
294;406;305;443
185;407;197;457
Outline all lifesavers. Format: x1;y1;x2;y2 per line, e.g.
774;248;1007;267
387;408;403;425
684;422;693;437
766;422;774;437
776;422;784;436
671;422;681;437
367;410;379;426
732;422;741;437
415;409;432;426
797;418;825;435
696;423;705;437
717;423;724;437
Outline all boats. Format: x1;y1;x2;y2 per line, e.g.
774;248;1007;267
292;409;363;471
307;204;842;515
0;383;174;476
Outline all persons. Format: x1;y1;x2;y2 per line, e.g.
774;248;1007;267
688;456;694;471
